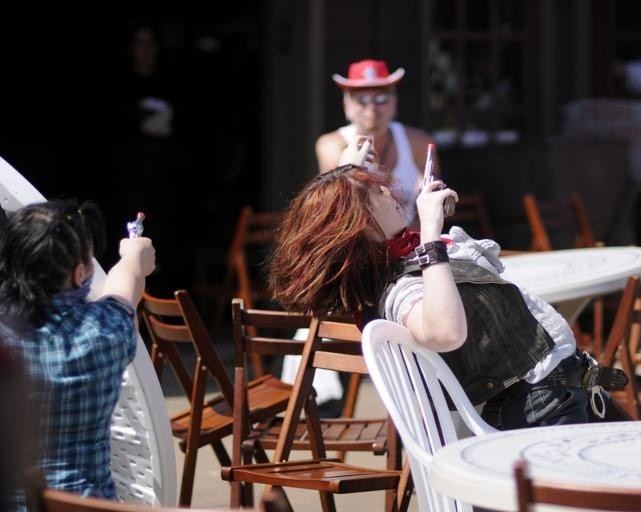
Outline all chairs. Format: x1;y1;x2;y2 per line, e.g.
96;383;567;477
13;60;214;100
512;458;640;511
136;289;306;510
228;298;402;512
220;312;416;511
26;467;237;510
361;317;506;512
228;195;641;420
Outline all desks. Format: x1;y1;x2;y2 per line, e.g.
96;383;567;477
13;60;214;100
425;418;641;512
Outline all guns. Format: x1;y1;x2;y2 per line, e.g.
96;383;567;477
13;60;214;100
420;143;455;216
126;213;144;238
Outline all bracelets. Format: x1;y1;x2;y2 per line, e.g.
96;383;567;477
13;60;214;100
413;240;451;272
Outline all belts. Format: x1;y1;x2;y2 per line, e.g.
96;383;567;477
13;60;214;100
505;351;629;396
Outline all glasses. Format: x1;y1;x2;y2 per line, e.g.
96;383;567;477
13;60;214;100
357;93;388;104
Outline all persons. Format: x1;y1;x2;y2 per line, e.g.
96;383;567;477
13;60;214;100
0;202;159;511
311;60;439;231
267;130;638;435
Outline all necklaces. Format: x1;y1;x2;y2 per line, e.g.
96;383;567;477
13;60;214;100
379;137;392;171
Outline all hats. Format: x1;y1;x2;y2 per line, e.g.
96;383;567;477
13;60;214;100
332;59;405;88
